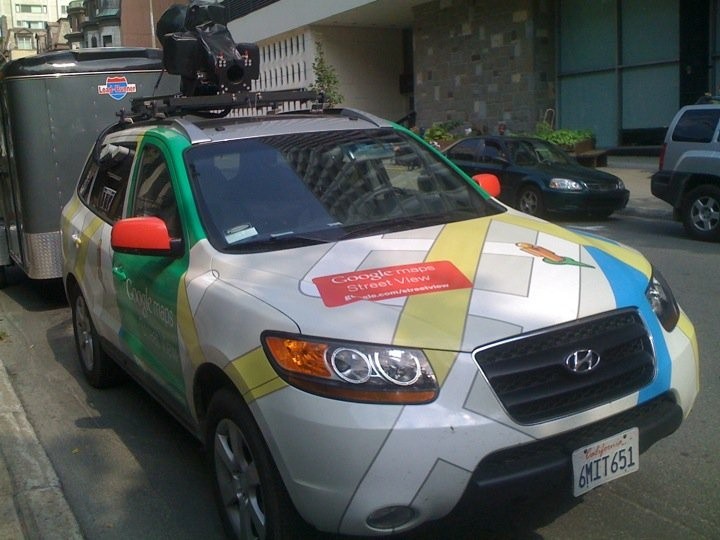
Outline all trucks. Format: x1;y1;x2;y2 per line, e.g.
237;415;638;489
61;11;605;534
1;49;184;307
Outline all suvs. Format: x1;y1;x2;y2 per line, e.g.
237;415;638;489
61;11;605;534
651;95;720;240
60;86;702;539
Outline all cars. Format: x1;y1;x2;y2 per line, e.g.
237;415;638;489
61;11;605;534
419;135;632;217
